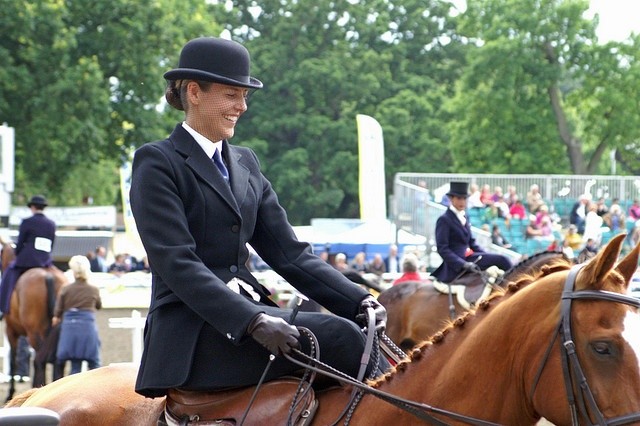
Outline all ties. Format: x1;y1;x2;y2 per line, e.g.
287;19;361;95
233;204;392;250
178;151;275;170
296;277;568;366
213;149;231;186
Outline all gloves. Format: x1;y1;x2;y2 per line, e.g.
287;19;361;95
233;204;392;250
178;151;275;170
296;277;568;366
249;312;302;359
358;297;390;337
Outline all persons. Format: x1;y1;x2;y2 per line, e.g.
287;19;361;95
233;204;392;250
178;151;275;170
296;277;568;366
431;183;514;281
604;214;613;227
538;217;551;237
495;195;511;218
92;245;107;272
548;238;559;252
353;252;368;271
483;223;489;231
583;238;601;252
385;245;401;272
628;227;640;249
110;253;151;272
393;256;431;285
616;214;631;234
370;254;388;274
559;239;574;258
53;255;101;380
493;186;503;203
419;181;435;201
467;185;479;207
630;199;640;218
335;253;348;270
534;206;548;224
526;185;544;213
129;38;389;399
321;252;329;261
564;224;582;248
524;215;537;240
610;198;622;214
503;186;519;201
596;197;609;215
1;196;55;321
583;205;603;236
481;184;493;206
490;225;513;250
509;200;526;219
570;194;589;233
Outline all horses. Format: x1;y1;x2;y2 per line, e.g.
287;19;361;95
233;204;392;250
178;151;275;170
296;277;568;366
0;236;69;402
377;250;574;350
0;231;640;426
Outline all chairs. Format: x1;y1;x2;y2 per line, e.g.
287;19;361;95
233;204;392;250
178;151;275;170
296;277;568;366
467;196;640;257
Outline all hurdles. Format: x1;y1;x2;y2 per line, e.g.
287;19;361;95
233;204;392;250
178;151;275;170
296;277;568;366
0;337;37;384
108;310;146;369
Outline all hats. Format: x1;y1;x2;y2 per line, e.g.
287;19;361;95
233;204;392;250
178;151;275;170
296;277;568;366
445;179;472;200
163;36;264;92
27;194;48;208
